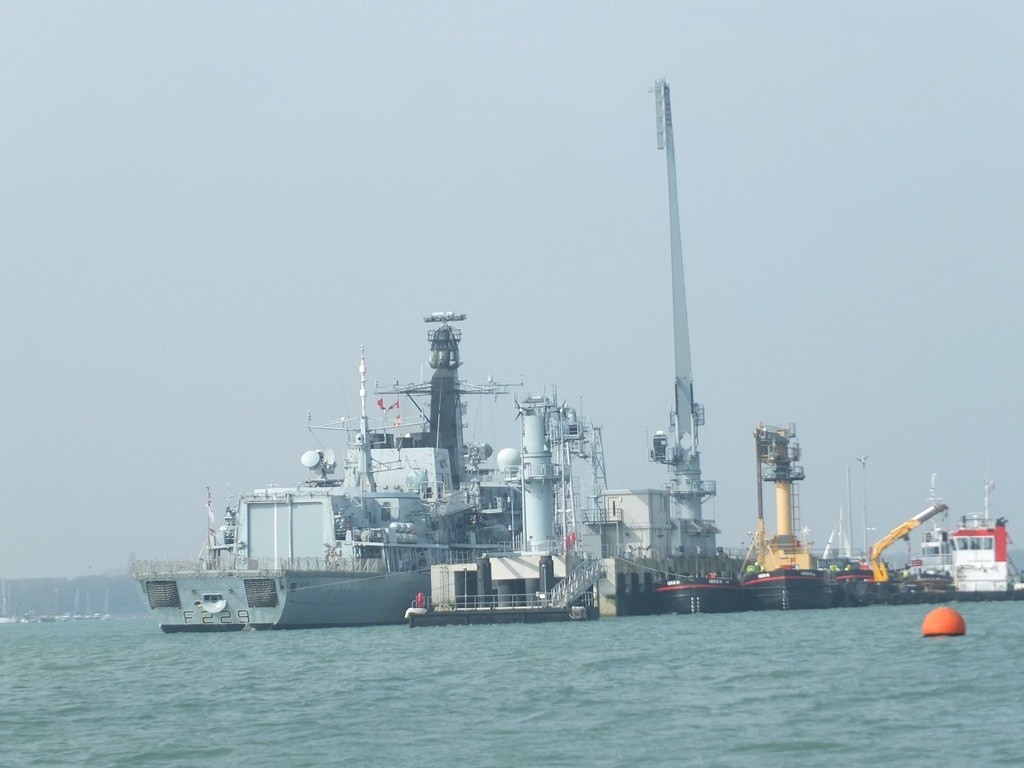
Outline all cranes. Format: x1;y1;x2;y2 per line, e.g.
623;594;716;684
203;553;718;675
646;76;723;560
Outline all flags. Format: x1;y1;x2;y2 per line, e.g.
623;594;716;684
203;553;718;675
377;399;399;410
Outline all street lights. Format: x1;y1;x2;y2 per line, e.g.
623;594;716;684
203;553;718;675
854;456;870;559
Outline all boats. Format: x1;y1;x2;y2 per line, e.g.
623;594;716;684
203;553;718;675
651;472;1024;612
132;312;610;634
